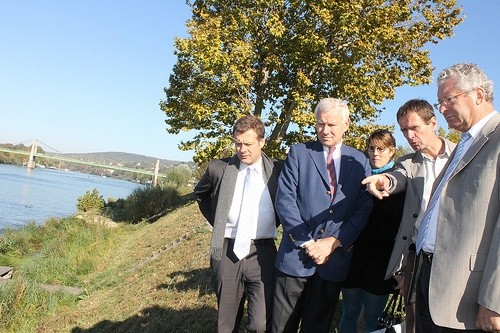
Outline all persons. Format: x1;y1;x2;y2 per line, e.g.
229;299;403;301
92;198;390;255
361;99;457;333
270;97;373;333
405;63;500;333
194;115;286;333
339;129;407;333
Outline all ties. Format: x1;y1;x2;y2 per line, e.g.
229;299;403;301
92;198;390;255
233;167;260;261
415;134;471;255
326;147;338;203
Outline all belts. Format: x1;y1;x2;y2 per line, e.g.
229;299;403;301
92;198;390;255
421;250;434;263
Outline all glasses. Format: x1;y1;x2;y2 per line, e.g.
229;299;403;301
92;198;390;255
366;145;387;154
433;88;475;110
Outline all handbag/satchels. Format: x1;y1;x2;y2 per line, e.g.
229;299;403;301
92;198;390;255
378;290;402;330
